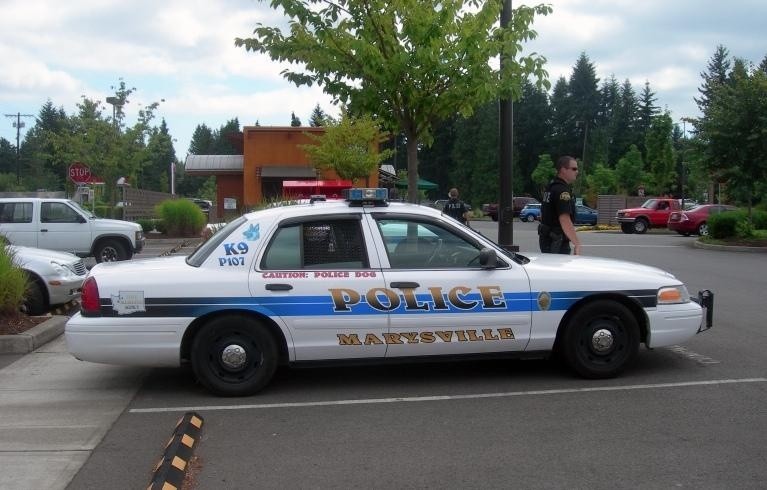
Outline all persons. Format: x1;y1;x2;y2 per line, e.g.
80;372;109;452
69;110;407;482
441;187;469;226
660;201;670;210
536;154;582;256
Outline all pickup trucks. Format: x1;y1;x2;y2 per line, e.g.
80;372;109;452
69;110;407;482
483;197;540;221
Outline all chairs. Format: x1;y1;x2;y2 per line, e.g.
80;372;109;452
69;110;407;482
327;226;348;260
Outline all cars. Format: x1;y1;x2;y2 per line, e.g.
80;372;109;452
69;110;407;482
3;245;90;316
64;186;714;398
433;199;471;211
615;198;741;237
520;202;598;226
200;194;437;252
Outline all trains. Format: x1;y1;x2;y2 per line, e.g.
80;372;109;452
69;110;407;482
74;185;213;224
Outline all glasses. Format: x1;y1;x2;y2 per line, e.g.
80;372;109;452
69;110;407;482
563;166;577;171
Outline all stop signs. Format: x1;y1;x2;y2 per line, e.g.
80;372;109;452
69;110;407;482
69;161;92;185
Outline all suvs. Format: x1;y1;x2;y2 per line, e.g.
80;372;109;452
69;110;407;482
0;197;146;263
